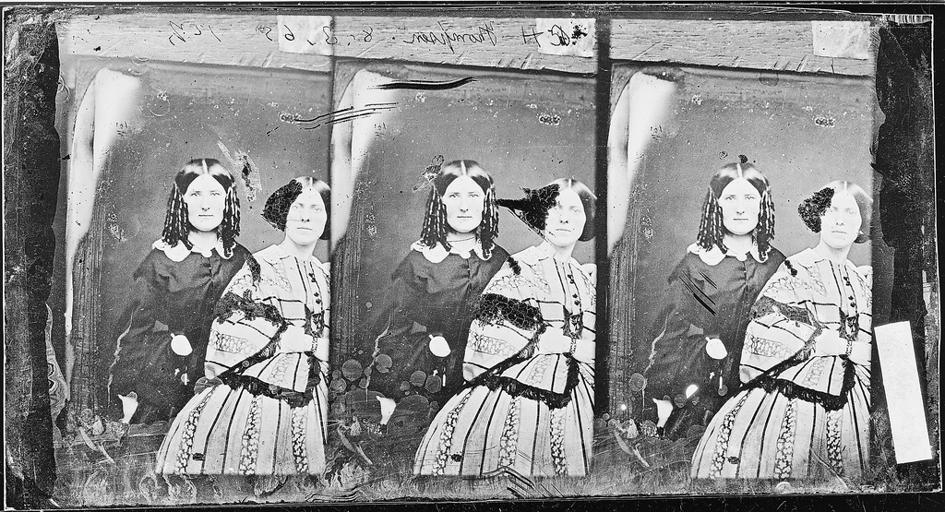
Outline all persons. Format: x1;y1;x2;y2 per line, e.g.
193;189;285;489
100;160;875;474
